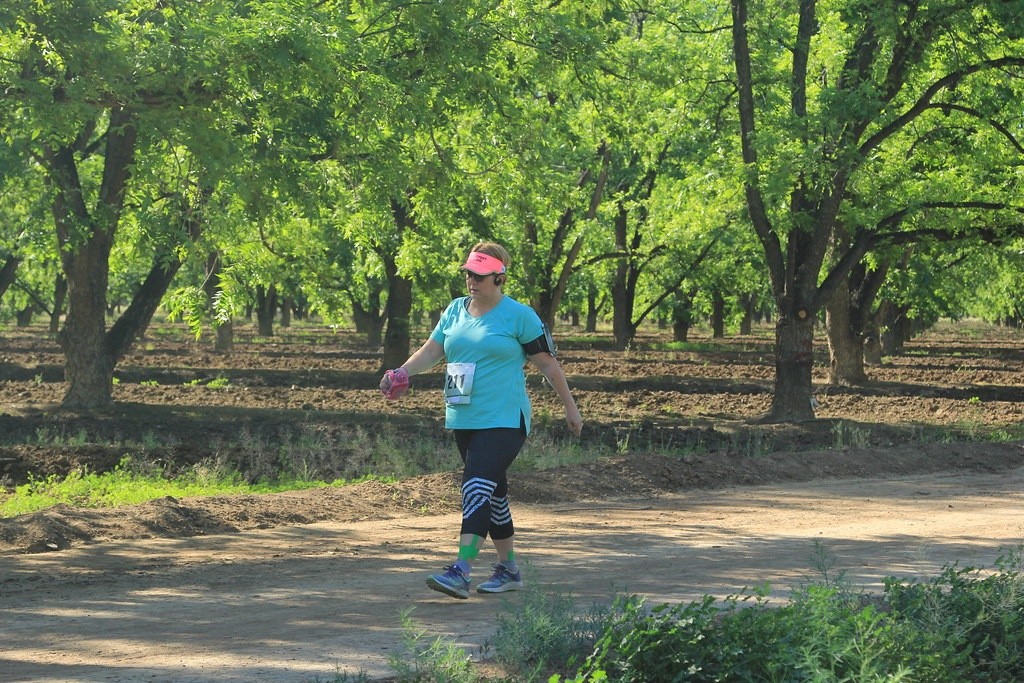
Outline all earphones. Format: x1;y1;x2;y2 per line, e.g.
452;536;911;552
494;275;502;286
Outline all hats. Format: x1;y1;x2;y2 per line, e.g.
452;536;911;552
462;251;506;276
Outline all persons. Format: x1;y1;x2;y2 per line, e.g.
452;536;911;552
379;241;583;599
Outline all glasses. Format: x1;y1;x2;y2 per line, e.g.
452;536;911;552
466;269;499;282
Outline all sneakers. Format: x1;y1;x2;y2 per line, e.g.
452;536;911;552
476;561;523;592
426;564;472;599
